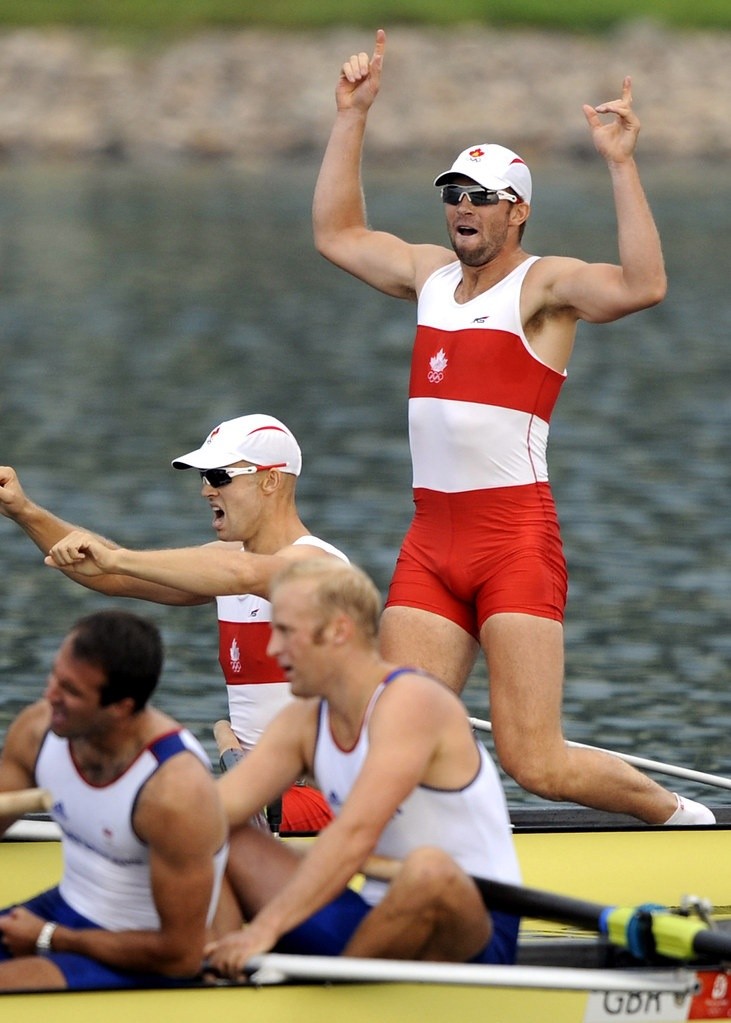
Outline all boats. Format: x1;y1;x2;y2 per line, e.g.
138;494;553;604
0;795;731;947
0;935;731;1023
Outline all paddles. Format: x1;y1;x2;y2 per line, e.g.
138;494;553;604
213;720;271;835
0;787;54;817
359;858;731;970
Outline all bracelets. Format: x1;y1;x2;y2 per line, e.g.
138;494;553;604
36;921;56;954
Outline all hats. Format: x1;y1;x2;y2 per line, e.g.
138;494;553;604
433;143;532;206
170;414;304;478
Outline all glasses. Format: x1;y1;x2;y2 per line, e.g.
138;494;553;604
194;462;287;488
439;183;523;207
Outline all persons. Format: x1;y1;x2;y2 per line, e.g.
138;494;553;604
0;609;227;993
311;27;715;823
1;412;347;830
205;554;519;993
